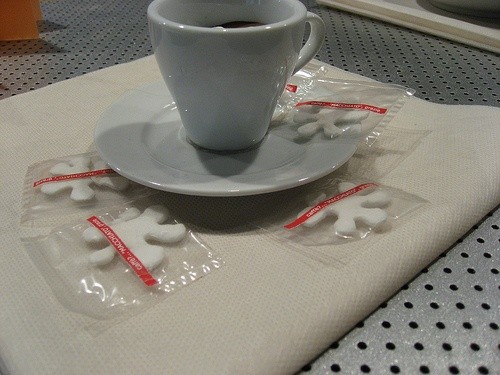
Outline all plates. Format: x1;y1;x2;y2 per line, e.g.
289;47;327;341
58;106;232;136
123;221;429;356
93;72;360;197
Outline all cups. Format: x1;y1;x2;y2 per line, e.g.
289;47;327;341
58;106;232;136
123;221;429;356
146;0;327;152
